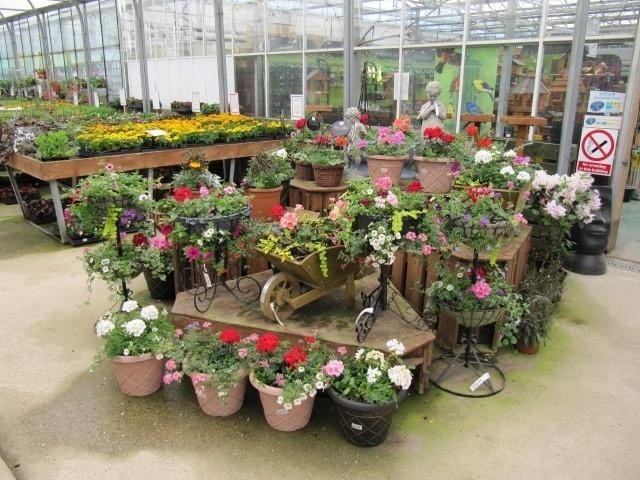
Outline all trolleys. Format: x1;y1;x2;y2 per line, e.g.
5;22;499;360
252;234;377;323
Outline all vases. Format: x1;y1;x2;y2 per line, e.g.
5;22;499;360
327;379;397;448
180;357;248;423
102;352;167;400
248;366;318;434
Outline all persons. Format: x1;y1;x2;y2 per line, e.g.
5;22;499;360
417;80;446;136
343;107;367;172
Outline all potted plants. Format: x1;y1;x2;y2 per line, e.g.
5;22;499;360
0;69;107;92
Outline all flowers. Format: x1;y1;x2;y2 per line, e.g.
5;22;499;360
326;337;418;408
164;318;260;410
237;334;342;413
0;97;602;346
85;300;178;389
